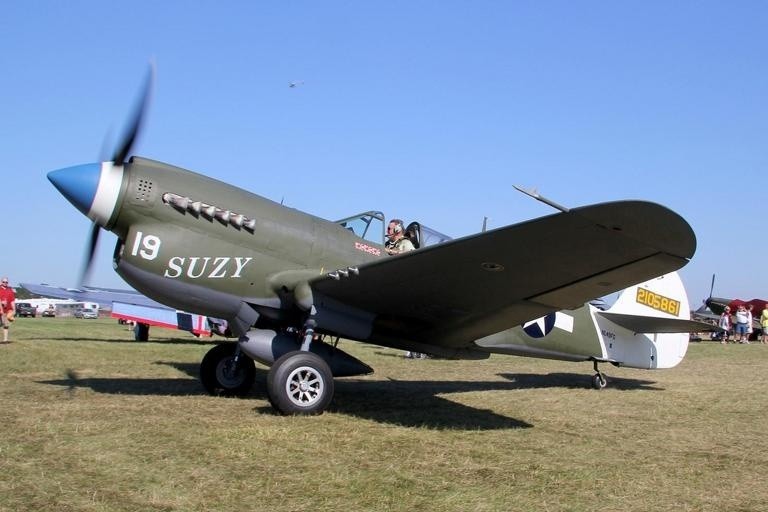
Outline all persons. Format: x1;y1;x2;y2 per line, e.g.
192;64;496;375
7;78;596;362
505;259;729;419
760;315;765;343
383;219;418;257
745;304;754;344
710;319;718;327
733;305;749;344
761;304;768;342
717;305;734;345
0;277;17;344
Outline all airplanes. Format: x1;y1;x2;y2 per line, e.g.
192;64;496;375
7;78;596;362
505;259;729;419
694;272;768;341
19;272;432;360
46;60;728;416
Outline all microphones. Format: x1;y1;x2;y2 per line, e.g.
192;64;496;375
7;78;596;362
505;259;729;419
385;234;393;237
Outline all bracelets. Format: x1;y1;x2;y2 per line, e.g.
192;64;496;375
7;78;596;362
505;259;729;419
390;249;392;254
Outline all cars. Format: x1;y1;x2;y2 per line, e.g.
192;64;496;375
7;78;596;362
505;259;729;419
73;308;97;320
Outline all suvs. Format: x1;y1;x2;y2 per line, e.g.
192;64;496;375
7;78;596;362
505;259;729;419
15;303;36;318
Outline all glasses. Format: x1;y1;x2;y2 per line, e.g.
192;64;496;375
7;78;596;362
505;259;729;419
388;226;396;230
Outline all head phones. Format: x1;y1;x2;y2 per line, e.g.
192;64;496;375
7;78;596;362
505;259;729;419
394;220;404;234
